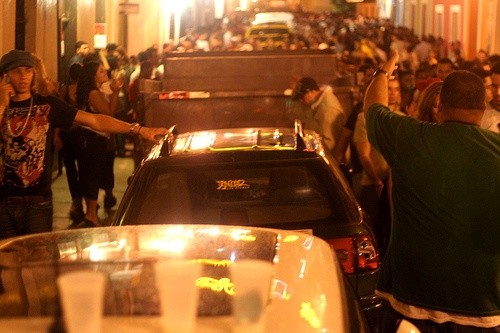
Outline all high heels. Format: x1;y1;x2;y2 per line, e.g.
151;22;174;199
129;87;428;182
85;216;102;226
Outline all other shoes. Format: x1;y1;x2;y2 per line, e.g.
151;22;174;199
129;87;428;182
97;203;99;210
104;196;116;208
70;211;84;221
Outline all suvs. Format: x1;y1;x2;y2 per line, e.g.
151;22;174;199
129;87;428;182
112;119;384;318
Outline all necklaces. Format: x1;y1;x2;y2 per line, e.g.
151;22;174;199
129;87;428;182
6;95;34;137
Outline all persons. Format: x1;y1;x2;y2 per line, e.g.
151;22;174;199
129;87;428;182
76;61;124;227
478;62;500;134
364;50;500;333
63;8;500;122
418;81;445;125
128;62;162;170
352;78;401;200
0;49;173;240
62;63;117;223
291;78;345;165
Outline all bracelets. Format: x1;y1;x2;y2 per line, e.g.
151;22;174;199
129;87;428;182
129;122;140;134
372;69;389;78
138;126;142;135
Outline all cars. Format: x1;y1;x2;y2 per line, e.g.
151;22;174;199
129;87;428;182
0;224;346;333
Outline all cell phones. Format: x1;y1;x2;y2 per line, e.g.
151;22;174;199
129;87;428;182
2;74;16;98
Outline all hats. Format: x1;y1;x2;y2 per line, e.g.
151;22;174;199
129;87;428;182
291;77;317;101
0;49;35;71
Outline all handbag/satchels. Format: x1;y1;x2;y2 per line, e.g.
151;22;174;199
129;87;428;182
71;104;110;142
